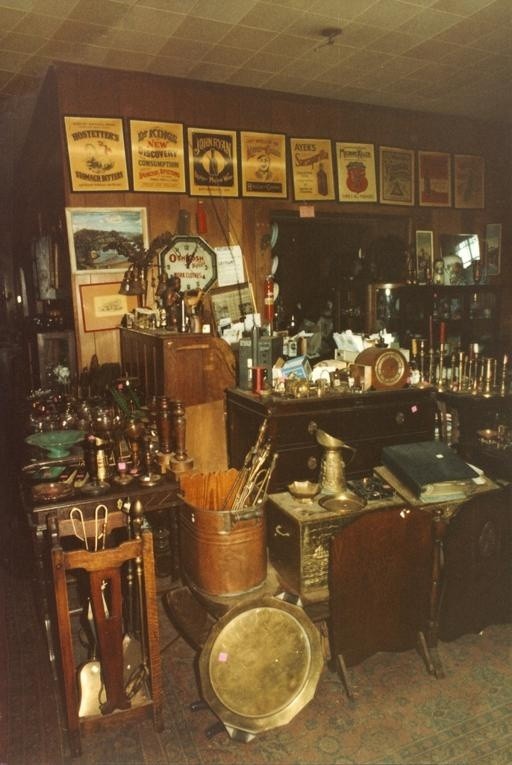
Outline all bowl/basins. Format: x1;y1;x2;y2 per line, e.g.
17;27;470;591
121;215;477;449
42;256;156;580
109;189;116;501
286;479;322;503
28;481;76;504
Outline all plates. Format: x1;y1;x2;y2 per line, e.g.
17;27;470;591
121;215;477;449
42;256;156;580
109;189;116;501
271;222;279;248
476;428;498;440
318;491;363;514
271;282;279;303
271;254;278;274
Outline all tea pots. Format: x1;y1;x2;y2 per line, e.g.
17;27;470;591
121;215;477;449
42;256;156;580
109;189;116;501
314;429;355;493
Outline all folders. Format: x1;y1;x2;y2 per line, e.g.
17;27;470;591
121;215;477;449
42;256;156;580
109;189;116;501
381;439;479;499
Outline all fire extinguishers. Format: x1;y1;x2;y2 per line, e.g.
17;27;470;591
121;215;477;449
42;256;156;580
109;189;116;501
263;275;275;324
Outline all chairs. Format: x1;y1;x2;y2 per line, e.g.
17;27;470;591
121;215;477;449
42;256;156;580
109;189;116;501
302;507;435;697
433;485;512;677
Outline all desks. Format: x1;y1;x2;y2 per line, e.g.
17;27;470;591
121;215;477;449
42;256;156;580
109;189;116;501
7;452;181;687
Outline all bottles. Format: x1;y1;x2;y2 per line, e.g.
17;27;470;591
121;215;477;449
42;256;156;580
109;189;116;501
178;298;187;332
190;305;201;334
433;258;444;286
208;147;218;175
274;295;301;360
424;262;432;281
196;200;208;233
252;365;354;401
432;286;499;323
149;313;157;330
317;162;328;196
410;337;485;392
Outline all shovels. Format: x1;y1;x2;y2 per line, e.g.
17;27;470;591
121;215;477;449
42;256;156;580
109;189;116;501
125;496;150;700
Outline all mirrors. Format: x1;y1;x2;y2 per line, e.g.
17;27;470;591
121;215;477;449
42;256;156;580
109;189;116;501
439;233;482;276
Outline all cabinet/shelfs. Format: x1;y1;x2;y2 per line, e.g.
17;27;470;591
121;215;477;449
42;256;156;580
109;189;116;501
267;476;504;626
225;383;432;496
120;327;240;477
331;274;510;355
431;388;511;480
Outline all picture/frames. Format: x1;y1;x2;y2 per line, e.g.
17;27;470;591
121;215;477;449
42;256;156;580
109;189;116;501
484;223;502;276
239;129;290;201
185;125;241;199
415;230;436;272
62;114;131;194
34;332;78;388
377;144;417;208
334;140;379;204
64;205;151;275
127;117;188;195
288;136;338;203
452;152;485;210
416;149;454;209
78;280;146;334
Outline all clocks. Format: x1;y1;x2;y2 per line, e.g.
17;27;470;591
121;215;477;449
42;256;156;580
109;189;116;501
158;235;218;297
